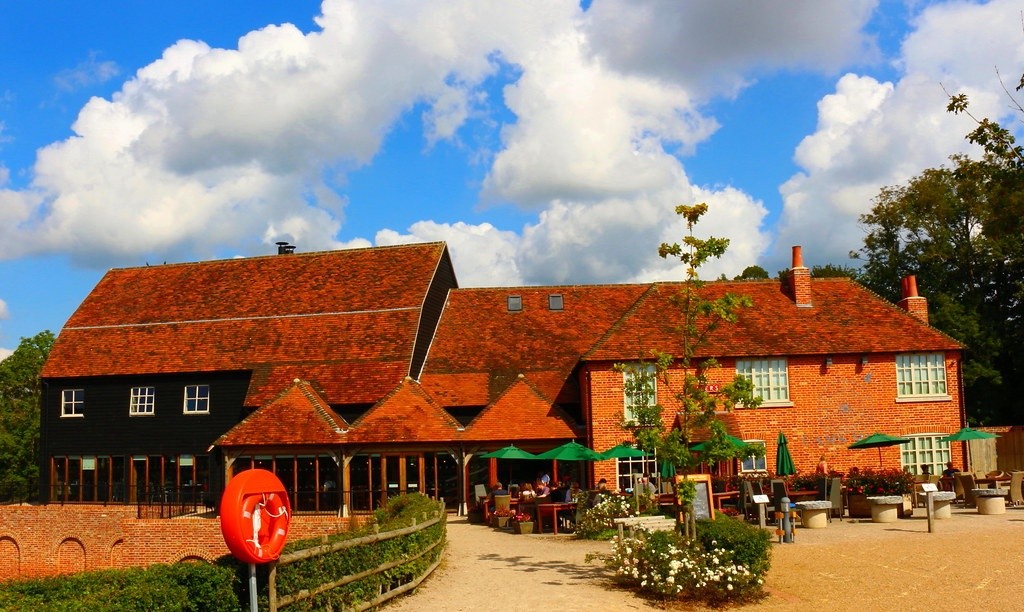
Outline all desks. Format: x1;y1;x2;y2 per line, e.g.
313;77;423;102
484;497;519;521
537;502;577;535
974;477;1011;489
656;490;822;511
939;476;955;491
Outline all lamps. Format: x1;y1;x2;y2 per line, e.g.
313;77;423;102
826;354;868;366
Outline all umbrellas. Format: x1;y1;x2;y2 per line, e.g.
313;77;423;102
848;433;910;467
661;459;676;493
690;435;748;452
479;438;655;493
937;427;1003;442
775;433;796;476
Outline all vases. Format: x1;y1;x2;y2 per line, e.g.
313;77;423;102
849;493;914;518
513;521;537;534
494;516;511;527
468;511;482;522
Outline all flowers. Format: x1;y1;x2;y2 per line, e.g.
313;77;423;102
494;505;532;522
469;503;481;513
847;467;914;496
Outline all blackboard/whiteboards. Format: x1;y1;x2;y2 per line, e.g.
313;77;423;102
674;475;716;523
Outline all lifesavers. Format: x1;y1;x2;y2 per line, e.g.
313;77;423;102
242;492;285;559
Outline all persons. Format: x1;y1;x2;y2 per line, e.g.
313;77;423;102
489;478;606;529
942;461;960;477
920;464;932;475
816;455;827;473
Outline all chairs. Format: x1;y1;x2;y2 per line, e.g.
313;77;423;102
747;482;756;514
663;481;673;493
724;481;747;519
753;481;775;524
911;471;1024;508
495;495;511;511
827;478;842;522
